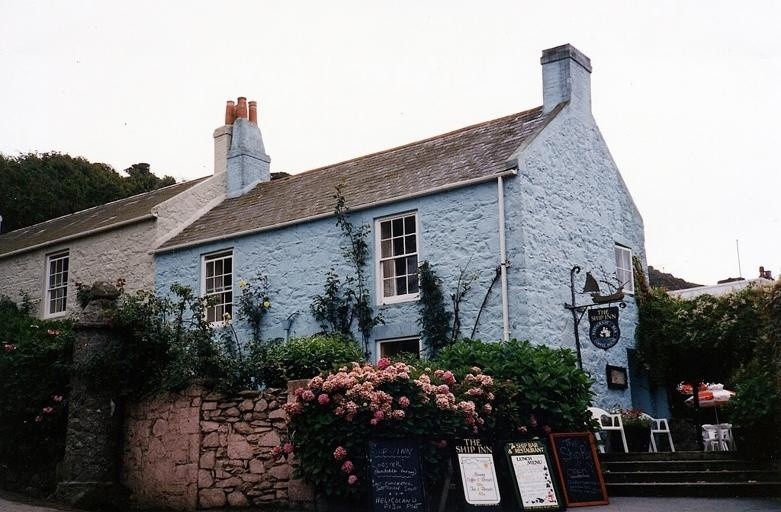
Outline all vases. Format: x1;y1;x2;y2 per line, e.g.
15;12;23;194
605;422;651;453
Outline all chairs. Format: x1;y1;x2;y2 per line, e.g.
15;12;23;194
586;407;628;451
701;424;733;452
637;413;672;452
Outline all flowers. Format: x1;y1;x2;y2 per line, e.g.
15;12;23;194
598;403;654;426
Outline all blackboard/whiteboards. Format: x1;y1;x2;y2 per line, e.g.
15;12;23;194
365;436;429;511
549;431;608;507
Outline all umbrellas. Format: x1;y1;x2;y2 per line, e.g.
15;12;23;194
683;382;741;426
